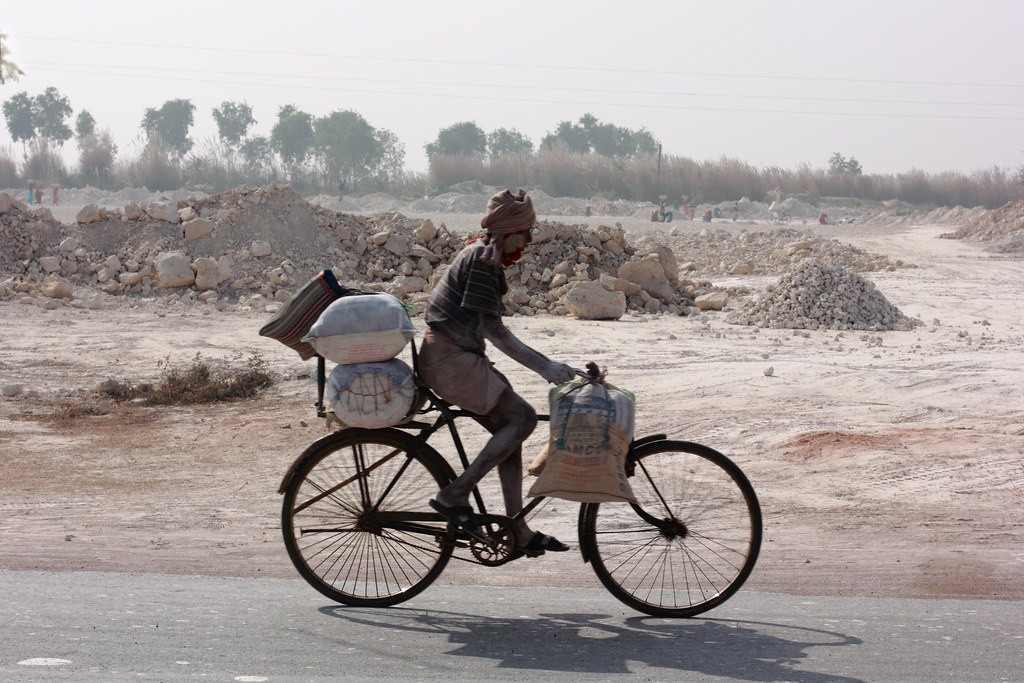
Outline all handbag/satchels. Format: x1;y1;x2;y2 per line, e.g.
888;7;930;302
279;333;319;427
258;269;352;362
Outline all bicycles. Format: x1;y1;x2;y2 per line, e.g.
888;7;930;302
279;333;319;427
768;210;792;225
275;362;765;618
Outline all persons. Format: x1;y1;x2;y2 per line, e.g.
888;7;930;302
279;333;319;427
25;185;59;206
584;196;855;225
417;189;576;551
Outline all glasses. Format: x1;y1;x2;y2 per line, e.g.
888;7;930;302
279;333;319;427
521;227;533;235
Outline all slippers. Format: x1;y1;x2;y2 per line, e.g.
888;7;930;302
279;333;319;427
506;530;570;552
429;498;491;546
